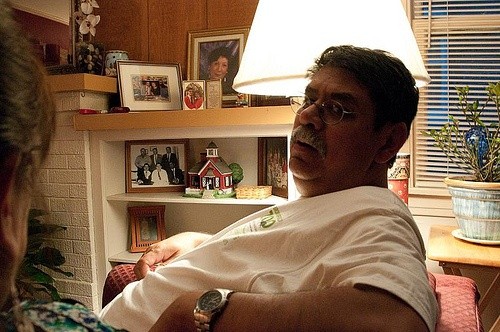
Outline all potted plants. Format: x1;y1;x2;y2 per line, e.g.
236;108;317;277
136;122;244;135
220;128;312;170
420;81;500;245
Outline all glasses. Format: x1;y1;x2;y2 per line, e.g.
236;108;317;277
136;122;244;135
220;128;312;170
189;91;194;92
289;96;374;125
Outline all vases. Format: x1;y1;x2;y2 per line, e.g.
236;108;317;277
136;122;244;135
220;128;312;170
77;41;105;75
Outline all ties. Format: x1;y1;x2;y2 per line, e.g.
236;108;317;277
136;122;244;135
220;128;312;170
173;169;174;173
191;96;193;104
155;155;156;161
168;155;169;160
158;171;161;180
146;172;147;173
147;88;148;94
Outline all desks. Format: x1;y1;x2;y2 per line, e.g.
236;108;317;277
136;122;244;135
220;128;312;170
428;224;500;332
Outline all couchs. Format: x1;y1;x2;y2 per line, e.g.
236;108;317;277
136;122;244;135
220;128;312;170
100;263;482;332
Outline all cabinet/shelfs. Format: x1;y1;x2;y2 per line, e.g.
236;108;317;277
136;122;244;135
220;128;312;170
73;104;302;306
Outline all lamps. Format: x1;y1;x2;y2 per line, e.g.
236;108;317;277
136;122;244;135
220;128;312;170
231;0;432;97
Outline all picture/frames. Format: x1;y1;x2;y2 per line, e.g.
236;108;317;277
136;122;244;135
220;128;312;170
180;80;206;110
186;24;252;108
114;58;184;113
258;136;288;199
126;204;166;253
124;140;191;193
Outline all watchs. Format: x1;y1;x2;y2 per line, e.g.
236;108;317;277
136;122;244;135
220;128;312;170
193;288;236;332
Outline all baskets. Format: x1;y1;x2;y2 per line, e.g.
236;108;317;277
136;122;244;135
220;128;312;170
235;185;272;199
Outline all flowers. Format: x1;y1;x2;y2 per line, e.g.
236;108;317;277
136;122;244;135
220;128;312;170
74;0;100;40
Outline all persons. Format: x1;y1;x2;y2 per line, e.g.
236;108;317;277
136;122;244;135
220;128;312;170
97;44;440;331
141;81;161;96
200;47;237;95
0;0;130;332
134;146;184;186
